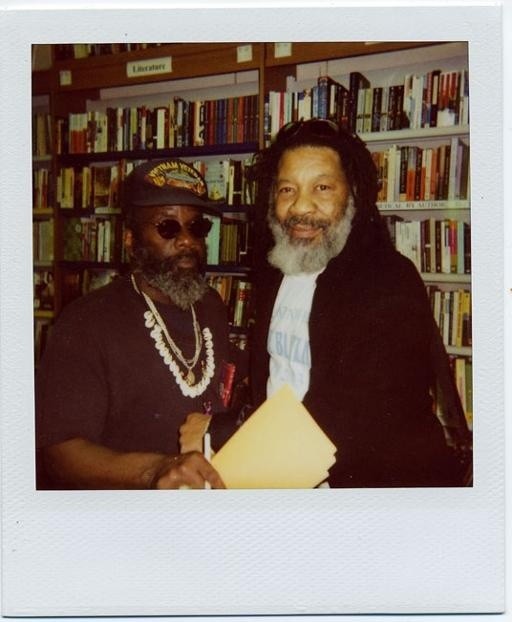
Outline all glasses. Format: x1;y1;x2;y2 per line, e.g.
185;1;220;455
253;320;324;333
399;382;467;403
135;216;214;240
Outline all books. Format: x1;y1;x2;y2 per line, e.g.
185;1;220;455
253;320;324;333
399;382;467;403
34;95;258;157
384;214;471;275
203;271;248;328
425;285;472;346
262;73;469;141
34;265;119;312
202;212;249;268
36;212;129;264
450;354;472;431
36;162;257;211
371;142;468;203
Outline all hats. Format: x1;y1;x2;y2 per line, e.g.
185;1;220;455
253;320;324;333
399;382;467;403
118;157;225;218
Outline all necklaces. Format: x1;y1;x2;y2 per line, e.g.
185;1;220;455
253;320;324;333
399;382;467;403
131;274;216;398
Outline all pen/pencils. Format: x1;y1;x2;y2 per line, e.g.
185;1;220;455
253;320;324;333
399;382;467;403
203;433;211;489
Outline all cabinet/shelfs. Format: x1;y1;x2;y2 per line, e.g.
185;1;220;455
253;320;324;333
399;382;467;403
32;42;473;446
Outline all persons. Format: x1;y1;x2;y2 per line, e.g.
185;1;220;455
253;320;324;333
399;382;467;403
180;116;457;488
35;161;249;490
